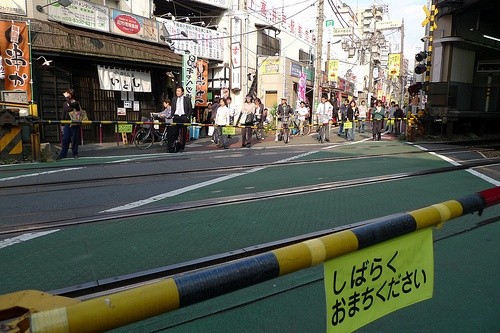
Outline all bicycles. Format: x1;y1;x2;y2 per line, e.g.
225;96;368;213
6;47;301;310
277;113;291;143
290;113;310;137
316;113;328;143
133;113;182;151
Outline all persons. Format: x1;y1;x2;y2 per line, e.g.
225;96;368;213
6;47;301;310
371;100;385;141
337;98;406;137
347;100;359;141
203;93;310;150
149;98;173;127
55;89;81;160
316;96;333;142
167;86;192;153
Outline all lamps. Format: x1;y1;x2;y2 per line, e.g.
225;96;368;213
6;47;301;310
408;81;428;96
172;47;190;54
191;21;205;26
175;16;190;21
160;12;172;18
166;70;179;78
160;31;198;43
36;0;71;12
36;56;53;66
415;63;428;75
415;50;429;61
421;37;428;42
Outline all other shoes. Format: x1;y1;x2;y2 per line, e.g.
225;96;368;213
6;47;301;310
72;156;78;158
240;145;245;148
246;144;250;147
56;156;65;160
325;139;329;142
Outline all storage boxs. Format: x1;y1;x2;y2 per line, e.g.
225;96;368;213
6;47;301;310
222;127;242;135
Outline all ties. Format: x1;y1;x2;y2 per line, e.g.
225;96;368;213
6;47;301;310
178;98;181;116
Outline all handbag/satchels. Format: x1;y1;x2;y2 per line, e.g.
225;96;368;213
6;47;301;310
69;102;88;127
240;112;247;124
